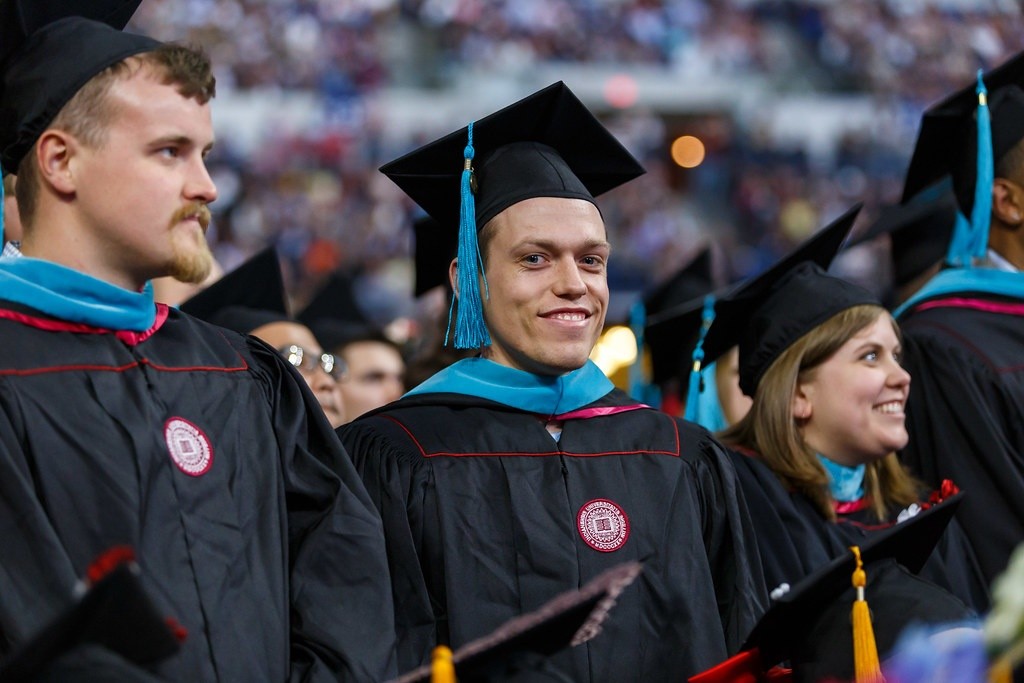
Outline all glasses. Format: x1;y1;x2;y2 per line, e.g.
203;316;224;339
278;343;353;386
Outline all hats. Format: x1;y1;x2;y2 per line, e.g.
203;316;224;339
740;490;985;682
395;589;617;682
632;251;720;326
177;243;297;335
287;261;400;351
687;205;885;435
900;52;1022;264
379;80;651;356
2;1;176;178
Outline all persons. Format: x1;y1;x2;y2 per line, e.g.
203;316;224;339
334;80;770;683
740;491;992;683
0;0;1024;430
891;52;1024;588
647;200;995;618
0;0;405;682
